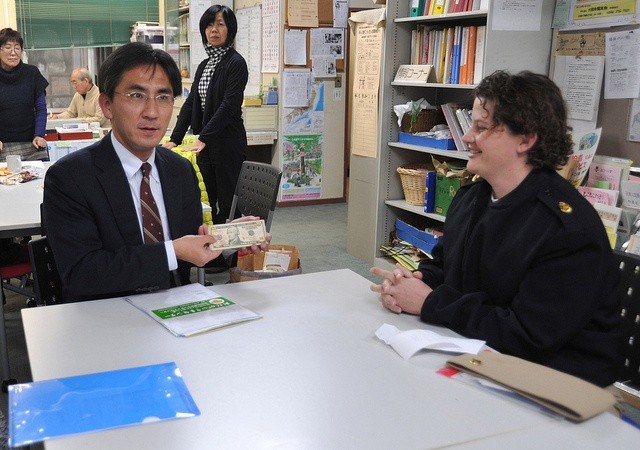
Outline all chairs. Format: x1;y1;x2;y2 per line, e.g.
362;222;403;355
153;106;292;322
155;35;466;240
1;243;33;307
225;161;283;242
612;250;639;389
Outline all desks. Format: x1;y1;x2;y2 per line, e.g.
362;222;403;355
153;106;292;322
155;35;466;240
1;161;211;285
20;269;640;450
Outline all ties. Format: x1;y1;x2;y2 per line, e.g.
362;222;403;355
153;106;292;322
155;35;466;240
140;164;165;243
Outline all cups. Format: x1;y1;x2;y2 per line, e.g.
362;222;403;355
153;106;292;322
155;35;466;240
6;156;23;173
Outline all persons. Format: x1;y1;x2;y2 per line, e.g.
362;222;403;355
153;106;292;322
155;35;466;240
0;28;49;160
370;70;627;390
227;225;243;247
160;5;249;224
45;45;270;300
48;68;110;128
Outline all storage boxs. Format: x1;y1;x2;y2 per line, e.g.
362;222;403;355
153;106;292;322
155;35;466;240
433;161;468;217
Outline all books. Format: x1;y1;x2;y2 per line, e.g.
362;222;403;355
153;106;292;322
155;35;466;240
440;104;474;151
179;14;189;44
380;218;442;271
245;106;276;129
179;48;190;75
567;128;640;254
410;24;487;84
410;1;476;16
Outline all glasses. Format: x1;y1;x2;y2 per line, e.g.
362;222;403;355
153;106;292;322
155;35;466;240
0;44;21;54
114;91;174;104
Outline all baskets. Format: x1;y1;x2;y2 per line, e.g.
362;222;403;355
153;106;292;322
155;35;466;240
397;162;437;206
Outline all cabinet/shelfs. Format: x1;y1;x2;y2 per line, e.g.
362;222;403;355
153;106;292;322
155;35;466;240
164;0;190;80
375;0;490;272
